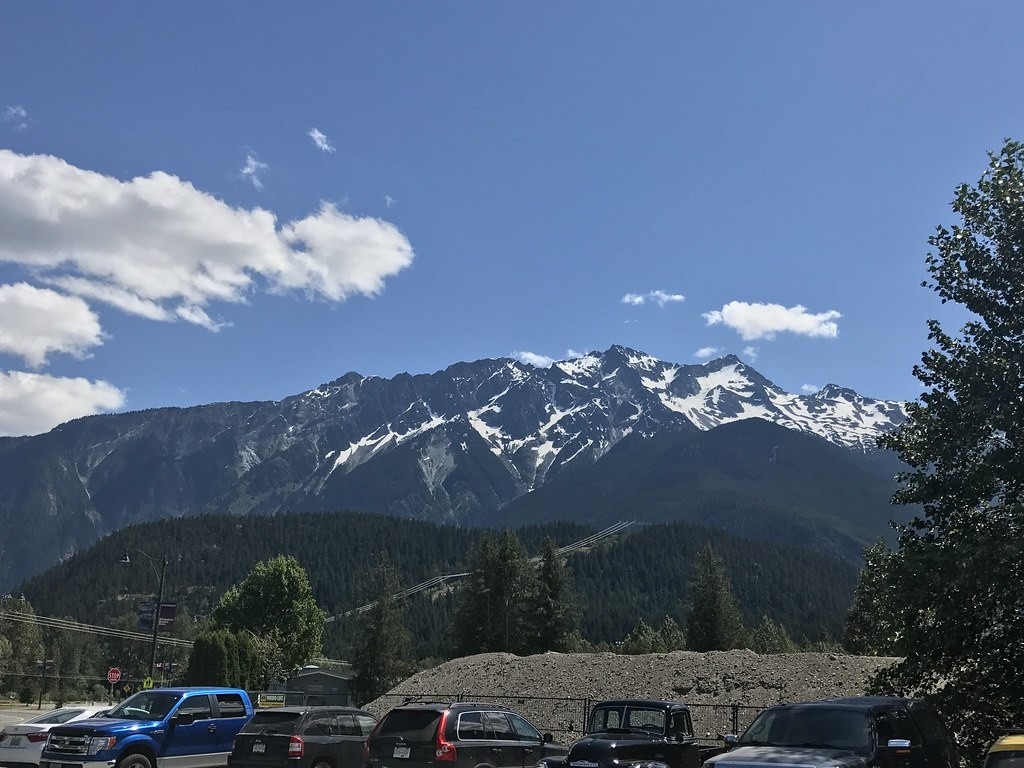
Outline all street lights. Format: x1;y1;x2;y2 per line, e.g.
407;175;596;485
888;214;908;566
107;668;121;683
120;550;168;711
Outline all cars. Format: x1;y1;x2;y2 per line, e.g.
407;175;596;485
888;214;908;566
0;706;150;768
983;727;1024;768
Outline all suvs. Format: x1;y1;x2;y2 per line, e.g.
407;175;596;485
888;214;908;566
227;706;380;768
39;687;255;768
702;696;961;768
362;700;569;768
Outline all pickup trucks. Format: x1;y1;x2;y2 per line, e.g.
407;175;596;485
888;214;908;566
534;700;731;768
